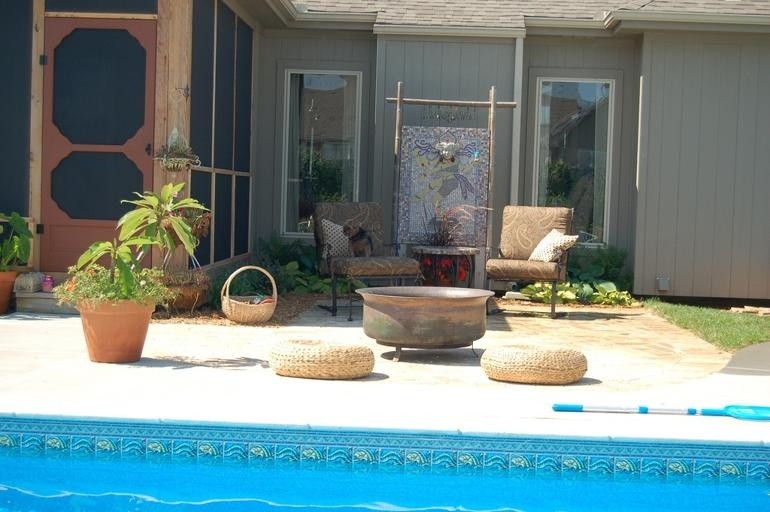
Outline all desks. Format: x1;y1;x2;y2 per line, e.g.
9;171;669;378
414;243;480;290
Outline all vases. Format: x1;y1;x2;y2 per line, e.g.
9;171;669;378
72;298;156;365
161;273;210;318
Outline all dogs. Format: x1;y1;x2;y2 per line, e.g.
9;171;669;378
343;219;373;257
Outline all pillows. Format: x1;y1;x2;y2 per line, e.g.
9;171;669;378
528;226;579;263
320;217;352;258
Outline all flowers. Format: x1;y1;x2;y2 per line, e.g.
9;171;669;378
50;235;179;309
108;182;213;291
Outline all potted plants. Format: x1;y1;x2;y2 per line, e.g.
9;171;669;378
0;209;35;315
153;136;202;178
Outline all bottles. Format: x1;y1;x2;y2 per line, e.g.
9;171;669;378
42;274;54;292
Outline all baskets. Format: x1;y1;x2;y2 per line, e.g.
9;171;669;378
221;266;277;323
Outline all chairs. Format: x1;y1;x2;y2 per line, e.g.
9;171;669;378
484;202;577;320
310;199;424;323
